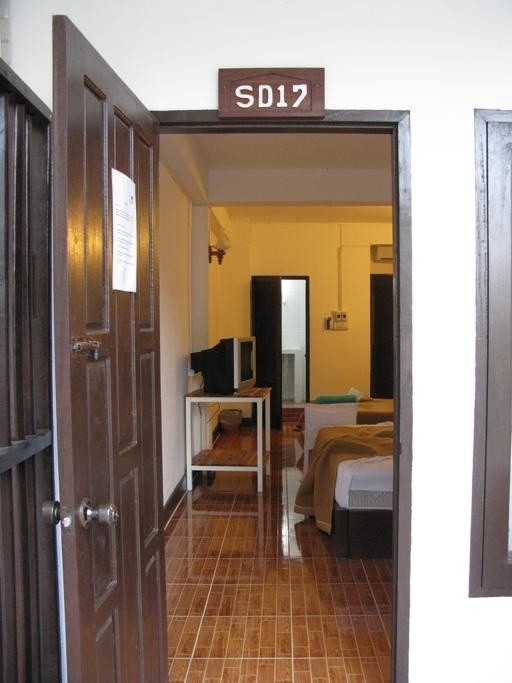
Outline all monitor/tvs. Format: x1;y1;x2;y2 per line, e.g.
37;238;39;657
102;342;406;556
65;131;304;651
190;336;258;395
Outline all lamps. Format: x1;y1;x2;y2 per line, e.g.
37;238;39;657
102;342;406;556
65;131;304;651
209;225;232;264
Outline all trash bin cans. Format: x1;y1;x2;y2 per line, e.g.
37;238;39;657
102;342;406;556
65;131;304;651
219;410;241;433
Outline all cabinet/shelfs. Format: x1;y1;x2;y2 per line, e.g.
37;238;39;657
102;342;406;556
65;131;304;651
183;386;272;493
186;490;273;587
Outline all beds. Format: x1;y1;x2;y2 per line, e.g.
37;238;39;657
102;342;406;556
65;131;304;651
304;396;394;421
304;424;395;556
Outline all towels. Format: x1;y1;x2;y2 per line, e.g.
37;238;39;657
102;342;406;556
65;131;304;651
317;394;355;404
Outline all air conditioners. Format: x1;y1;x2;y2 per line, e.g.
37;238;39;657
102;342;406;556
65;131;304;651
370;244;394;263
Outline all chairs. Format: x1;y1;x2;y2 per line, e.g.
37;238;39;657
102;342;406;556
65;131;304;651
303;403;357;476
